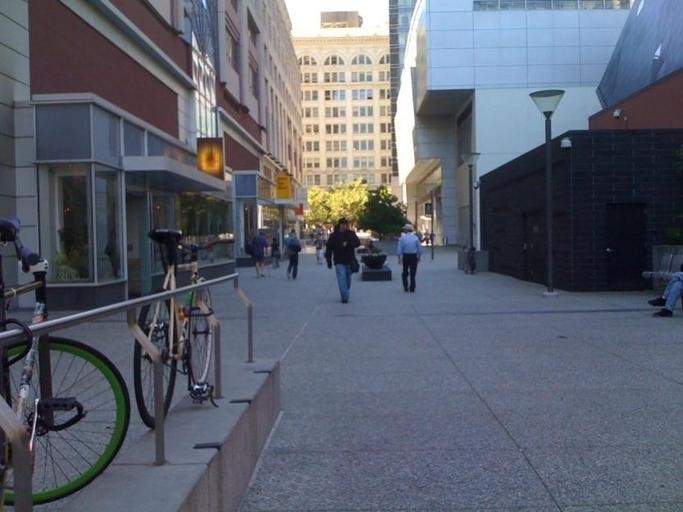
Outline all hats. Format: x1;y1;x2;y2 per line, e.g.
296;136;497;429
401;224;412;231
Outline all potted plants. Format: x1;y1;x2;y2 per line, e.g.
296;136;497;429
359;247;387;269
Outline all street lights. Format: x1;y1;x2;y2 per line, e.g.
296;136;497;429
460;152;481;274
530;90;566;293
413;182;439;260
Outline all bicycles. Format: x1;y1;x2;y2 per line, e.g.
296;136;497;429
0;216;132;506
134;230;235;429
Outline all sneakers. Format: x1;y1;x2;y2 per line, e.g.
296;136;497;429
648;296;666;306
653;308;672;317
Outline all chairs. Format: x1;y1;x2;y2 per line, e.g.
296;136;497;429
642;254;683;281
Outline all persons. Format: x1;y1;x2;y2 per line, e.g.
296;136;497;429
286;233;301;279
326;219;361;303
397;224;421;292
424;229;430;246
313;233;325;264
272;238;281;268
252;232;269;278
648;263;683;317
